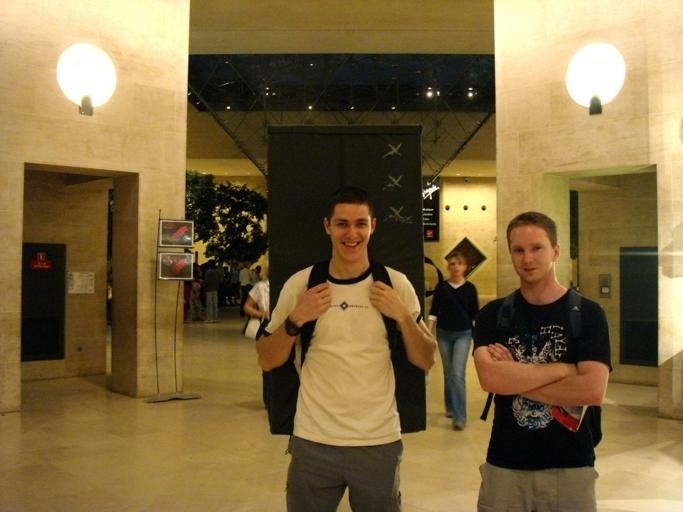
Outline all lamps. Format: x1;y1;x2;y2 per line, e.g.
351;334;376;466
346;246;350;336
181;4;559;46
53;39;120;116
564;41;631;117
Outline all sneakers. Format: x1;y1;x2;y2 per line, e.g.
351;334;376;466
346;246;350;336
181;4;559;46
446;411;466;430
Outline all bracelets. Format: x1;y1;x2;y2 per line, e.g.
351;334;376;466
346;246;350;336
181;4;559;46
285;316;302;336
574;361;580;374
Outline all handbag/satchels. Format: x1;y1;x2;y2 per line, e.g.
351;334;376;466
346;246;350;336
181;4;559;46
242;316;263;341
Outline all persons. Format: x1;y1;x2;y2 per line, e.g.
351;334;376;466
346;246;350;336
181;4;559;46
255;184;437;512
472;211;613;512
183;256;262;323
242;270;270;410
427;249;481;432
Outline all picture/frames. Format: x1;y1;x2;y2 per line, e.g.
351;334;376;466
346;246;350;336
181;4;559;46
157;252;194;281
158;219;196;249
